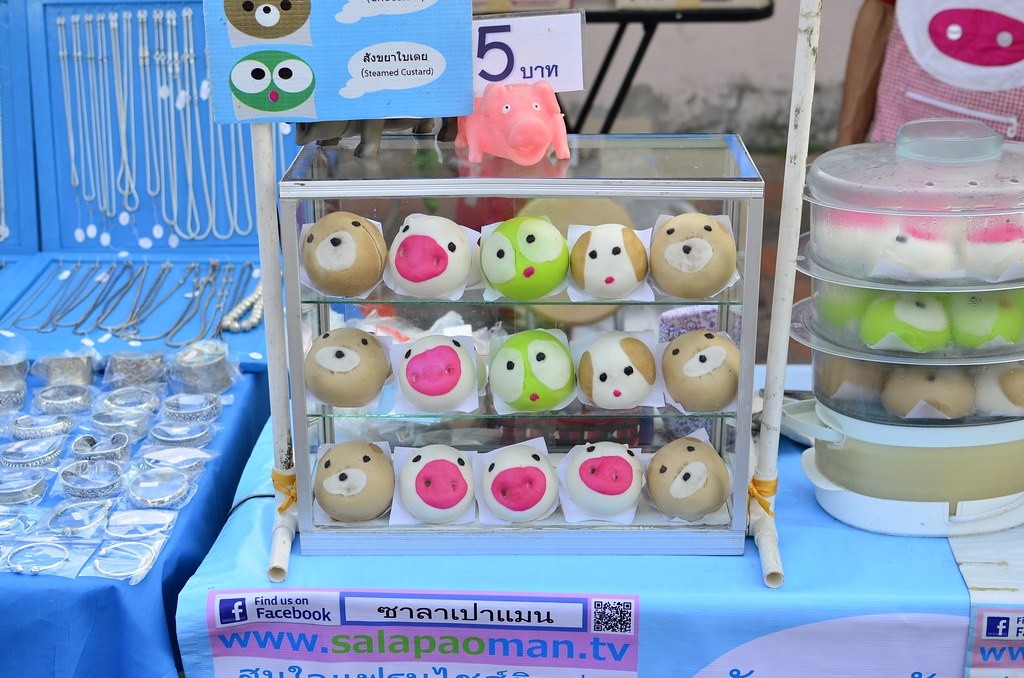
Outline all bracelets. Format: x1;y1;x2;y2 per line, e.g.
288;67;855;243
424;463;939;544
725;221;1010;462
0;340;231;576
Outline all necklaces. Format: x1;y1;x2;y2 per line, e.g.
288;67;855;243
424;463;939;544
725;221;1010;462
57;9;252;240
0;262;264;347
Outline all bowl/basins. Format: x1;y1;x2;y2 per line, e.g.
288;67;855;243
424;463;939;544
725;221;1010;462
786;119;1024;538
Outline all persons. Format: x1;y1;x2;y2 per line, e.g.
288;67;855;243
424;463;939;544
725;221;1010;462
836;0;1024;149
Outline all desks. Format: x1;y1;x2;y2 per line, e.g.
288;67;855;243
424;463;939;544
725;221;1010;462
473;0;776;183
175;356;1024;678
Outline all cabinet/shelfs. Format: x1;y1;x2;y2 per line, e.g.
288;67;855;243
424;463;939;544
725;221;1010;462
282;133;767;559
